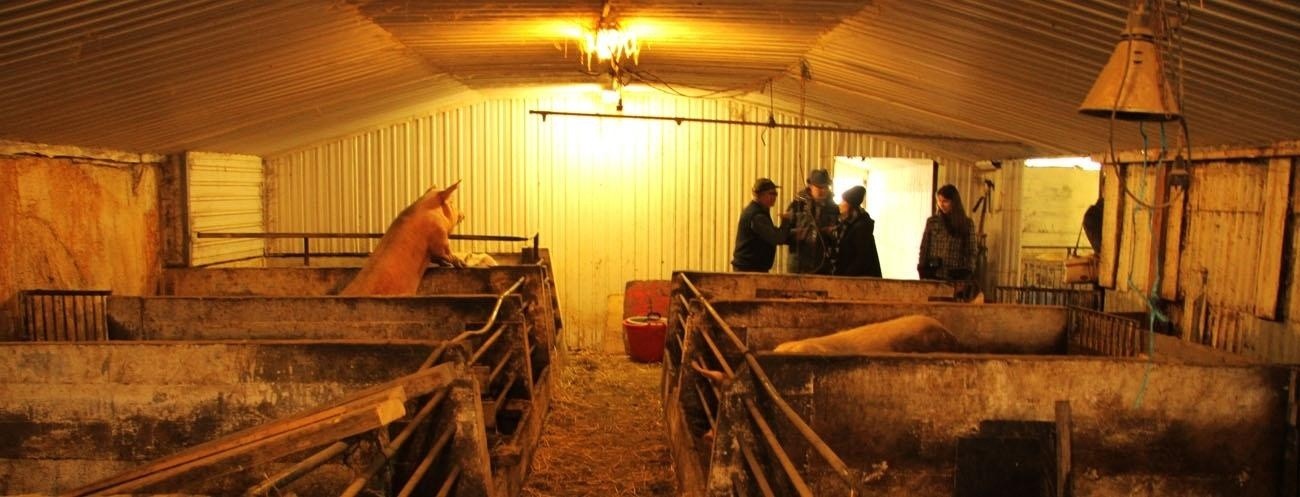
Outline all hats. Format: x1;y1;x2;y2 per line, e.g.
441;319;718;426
842;186;866;205
806;168;833;188
752;178;783;193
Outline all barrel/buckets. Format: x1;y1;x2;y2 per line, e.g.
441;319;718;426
621;313;667;363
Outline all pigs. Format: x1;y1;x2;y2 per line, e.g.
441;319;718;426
690;314;962;449
337;178;466;297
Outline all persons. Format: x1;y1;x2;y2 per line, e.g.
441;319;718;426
785;168;841;275
731;176;794;273
916;182;981;281
835;184;883;277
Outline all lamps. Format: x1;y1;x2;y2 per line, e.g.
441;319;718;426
1075;1;1184;123
